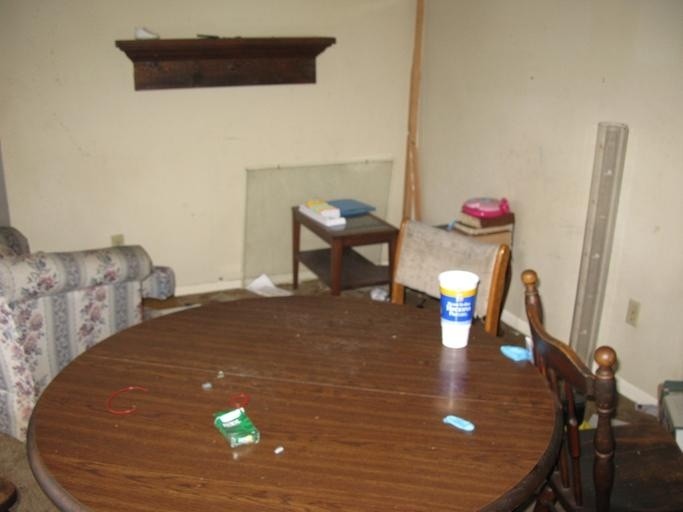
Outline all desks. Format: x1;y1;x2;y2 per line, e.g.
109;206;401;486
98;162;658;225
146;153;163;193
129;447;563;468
291;206;400;300
27;294;564;512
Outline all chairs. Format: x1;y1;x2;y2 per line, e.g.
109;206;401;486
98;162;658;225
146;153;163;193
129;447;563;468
390;217;510;338
520;268;683;512
0;225;175;443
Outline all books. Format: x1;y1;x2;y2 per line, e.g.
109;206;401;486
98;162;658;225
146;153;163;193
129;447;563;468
305;198;376;218
460;211;514;228
453;220;513;236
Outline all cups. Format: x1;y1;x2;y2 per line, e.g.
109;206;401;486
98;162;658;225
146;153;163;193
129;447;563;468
438;269;481;349
